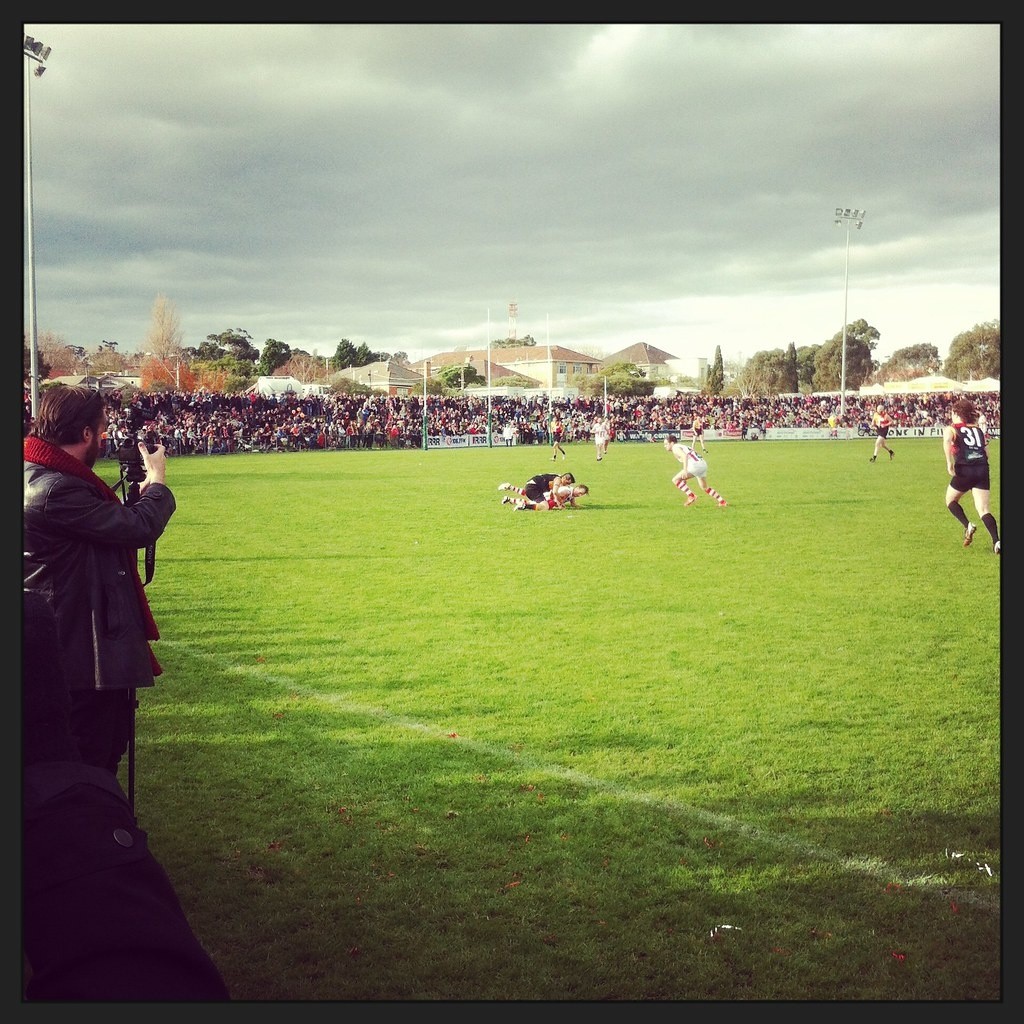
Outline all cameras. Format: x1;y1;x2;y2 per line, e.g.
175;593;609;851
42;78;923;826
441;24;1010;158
115;407;161;482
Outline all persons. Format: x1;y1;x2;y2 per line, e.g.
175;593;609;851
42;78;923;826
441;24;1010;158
518;473;574;511
944;400;1001;552
501;482;592;509
177;390;999;463
25;385;176;781
18;592;228;1002
664;433;726;505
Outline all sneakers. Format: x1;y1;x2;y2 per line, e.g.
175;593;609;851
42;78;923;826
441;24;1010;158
684;495;698;506
502;496;510;504
514;500;525;511
498;483;511;491
962;522;976;547
994;540;1000;554
